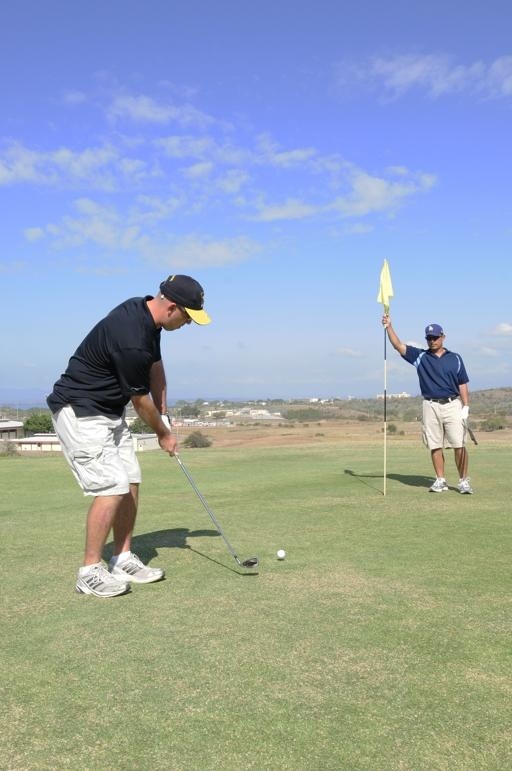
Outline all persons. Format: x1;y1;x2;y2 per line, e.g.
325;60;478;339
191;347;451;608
382;312;474;495
45;273;211;597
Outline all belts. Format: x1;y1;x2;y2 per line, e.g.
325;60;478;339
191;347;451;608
425;395;457;403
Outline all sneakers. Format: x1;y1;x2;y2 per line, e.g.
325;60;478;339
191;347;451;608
430;478;448;492
108;553;165;583
76;564;130;597
458;479;473;493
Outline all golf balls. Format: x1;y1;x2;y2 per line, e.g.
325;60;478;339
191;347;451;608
277;549;286;559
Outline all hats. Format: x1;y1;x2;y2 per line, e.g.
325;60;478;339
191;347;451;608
425;324;443;337
160;275;212;325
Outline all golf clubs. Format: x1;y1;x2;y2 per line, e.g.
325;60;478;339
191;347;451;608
173;450;259;568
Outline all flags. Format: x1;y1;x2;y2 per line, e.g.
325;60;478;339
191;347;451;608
375;261;395;310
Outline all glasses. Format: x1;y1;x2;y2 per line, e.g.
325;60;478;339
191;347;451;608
176;304;192;320
426;336;439;340
